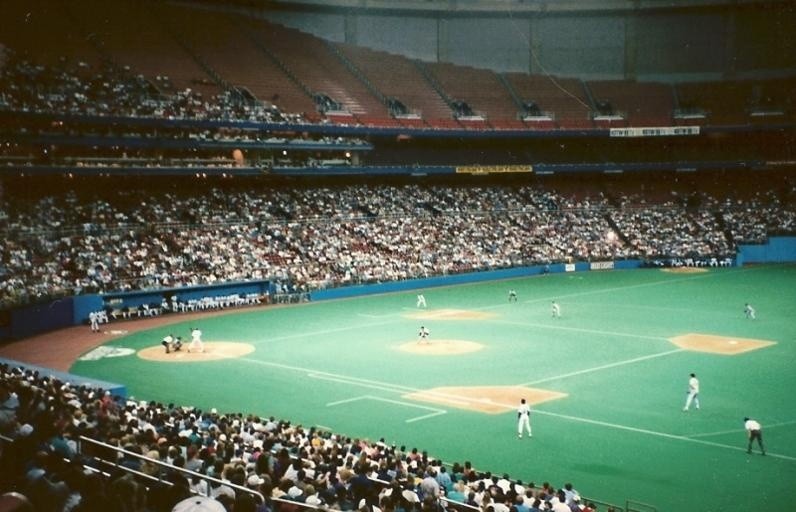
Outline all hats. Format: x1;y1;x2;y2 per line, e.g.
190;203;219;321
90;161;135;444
173;496;227;512
4;396;19;408
287;486;321;506
248;474;265;485
20;423;34;437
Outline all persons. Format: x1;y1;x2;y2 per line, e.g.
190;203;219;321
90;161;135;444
23;8;47;25
173;336;182;351
162;334;173;353
417;326;430;345
1;30;796;332
744;417;765;455
187;328;205;352
0;361;617;511
682;373;700;411
